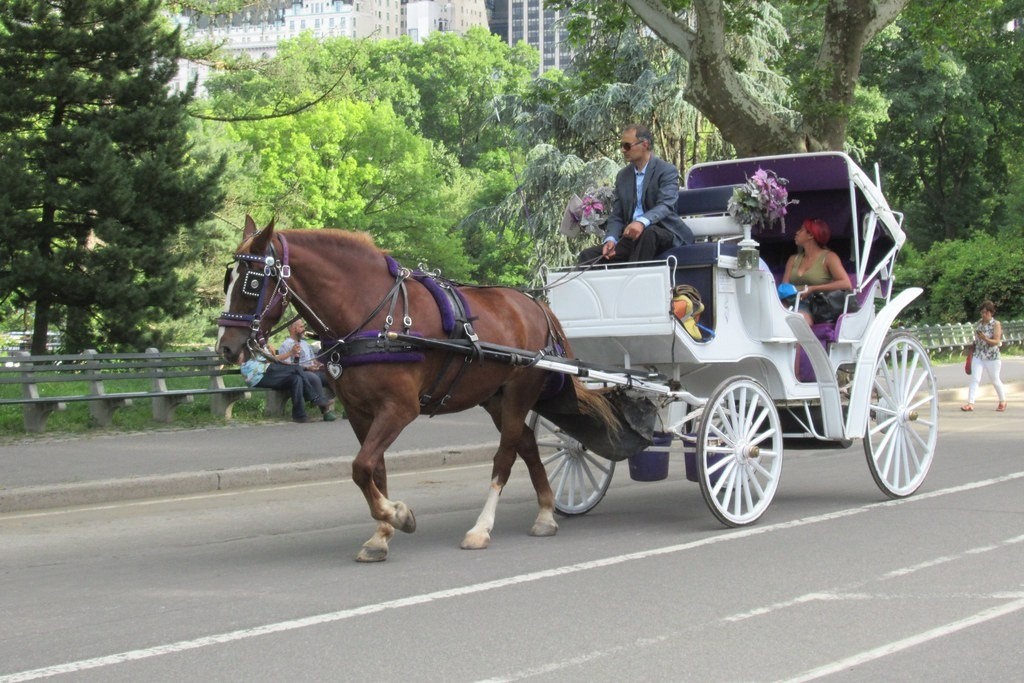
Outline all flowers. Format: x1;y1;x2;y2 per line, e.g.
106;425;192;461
568;186;616;240
727;169;801;234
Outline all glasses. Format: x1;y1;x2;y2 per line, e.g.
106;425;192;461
621;140;644;150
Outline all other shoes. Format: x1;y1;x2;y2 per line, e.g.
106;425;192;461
294;415;312;422
311;396;335;407
996;401;1007;410
961;405;973;410
324;410;336;420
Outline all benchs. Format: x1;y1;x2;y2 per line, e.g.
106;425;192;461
0;346;293;435
768;271;885;382
655;182;744;341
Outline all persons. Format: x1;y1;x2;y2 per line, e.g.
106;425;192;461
239;318;347;422
887;322;918;350
780;219;853;383
574;124;695;269
959;300;1008;412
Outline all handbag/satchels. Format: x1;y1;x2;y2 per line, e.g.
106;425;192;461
965;346;971;374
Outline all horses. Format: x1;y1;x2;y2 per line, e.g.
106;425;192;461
214;213;624;562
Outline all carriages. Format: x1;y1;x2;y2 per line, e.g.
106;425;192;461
213;149;940;563
